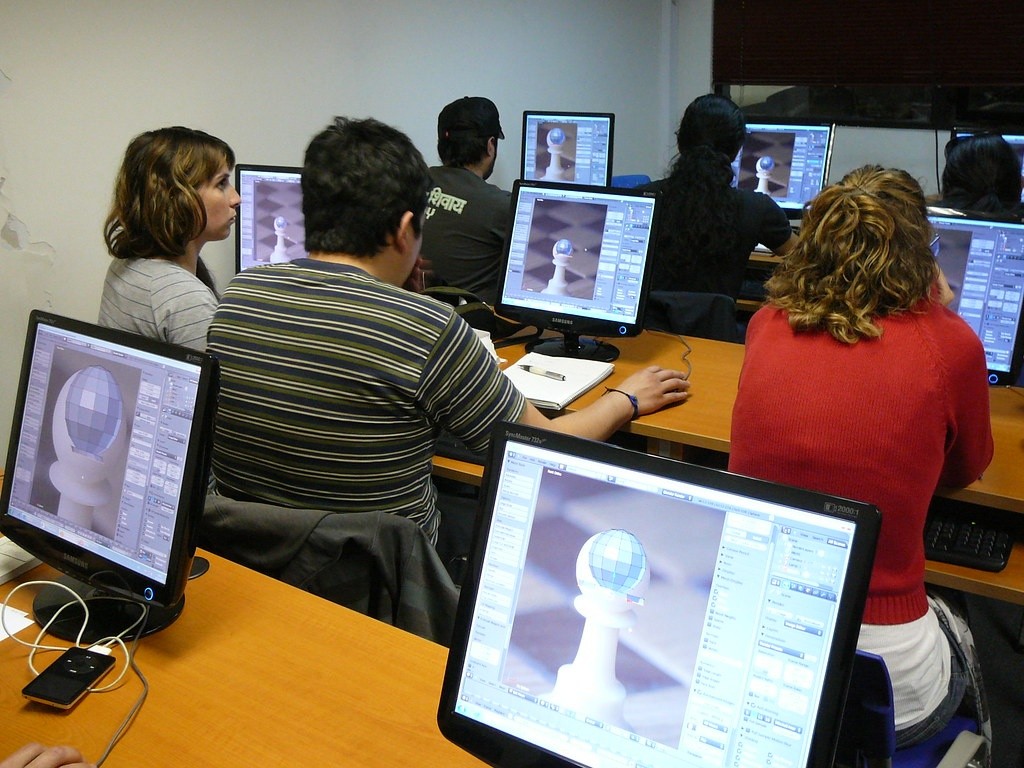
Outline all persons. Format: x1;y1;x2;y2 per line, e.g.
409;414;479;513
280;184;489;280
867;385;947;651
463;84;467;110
936;131;1024;386
630;94;798;342
418;96;512;305
204;116;691;586
97;125;242;353
727;164;993;768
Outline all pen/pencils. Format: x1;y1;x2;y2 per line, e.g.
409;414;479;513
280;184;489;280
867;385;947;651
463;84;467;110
517;364;566;381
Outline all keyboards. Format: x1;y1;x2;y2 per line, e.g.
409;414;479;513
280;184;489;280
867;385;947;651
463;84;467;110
738;268;775;300
924;495;1018;572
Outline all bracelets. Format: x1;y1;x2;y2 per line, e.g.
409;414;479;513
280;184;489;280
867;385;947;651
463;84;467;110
601;386;638;421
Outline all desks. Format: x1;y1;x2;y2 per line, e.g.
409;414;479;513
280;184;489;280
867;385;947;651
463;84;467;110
484;320;1024;606
0;471;491;768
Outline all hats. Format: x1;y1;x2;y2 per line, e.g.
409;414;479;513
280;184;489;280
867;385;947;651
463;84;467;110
439;96;505;139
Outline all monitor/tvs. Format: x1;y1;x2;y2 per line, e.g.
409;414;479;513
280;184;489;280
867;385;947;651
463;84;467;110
728;113;836;220
493;179;664;363
0;307;222;645
436;421;881;767
234;164;309;275
922;213;1024;387
949;126;1023;208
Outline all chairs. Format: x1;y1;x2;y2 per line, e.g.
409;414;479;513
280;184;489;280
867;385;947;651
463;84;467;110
836;650;990;768
